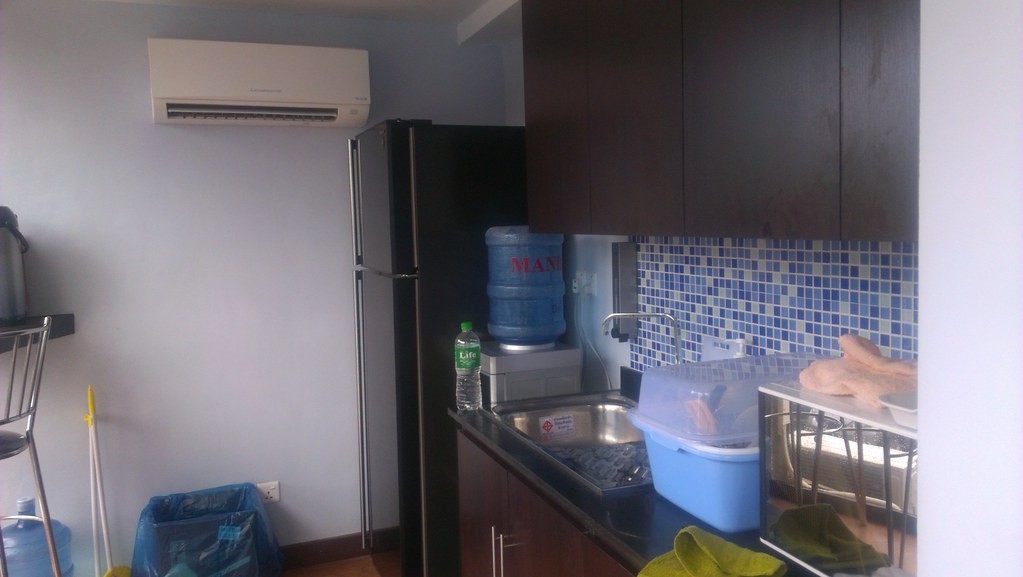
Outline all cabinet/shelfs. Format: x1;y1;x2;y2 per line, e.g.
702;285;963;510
521;0;922;240
455;427;637;577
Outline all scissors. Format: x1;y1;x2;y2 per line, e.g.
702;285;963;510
683;399;721;449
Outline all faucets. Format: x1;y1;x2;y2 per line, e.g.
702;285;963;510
599;310;688;365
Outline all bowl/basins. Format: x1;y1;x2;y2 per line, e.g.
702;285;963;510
879;391;918;429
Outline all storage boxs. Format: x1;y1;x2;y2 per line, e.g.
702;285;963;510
624;348;844;536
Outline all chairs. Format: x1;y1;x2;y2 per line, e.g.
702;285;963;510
0;317;64;577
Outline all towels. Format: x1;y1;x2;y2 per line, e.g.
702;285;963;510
800;331;917;409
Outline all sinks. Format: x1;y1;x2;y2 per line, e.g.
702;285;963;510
477;388;679;498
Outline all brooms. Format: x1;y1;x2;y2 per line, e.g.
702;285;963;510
86;382;134;577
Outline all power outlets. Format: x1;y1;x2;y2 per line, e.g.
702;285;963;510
573;269;598;297
254;479;281;505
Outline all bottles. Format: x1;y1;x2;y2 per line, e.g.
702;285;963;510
1;497;76;577
485;224;566;345
454;321;483;411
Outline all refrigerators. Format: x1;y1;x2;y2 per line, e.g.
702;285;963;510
346;114;532;577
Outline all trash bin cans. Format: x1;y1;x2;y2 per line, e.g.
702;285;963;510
131;482;283;576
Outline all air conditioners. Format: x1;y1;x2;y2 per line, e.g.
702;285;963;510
146;37;370;129
